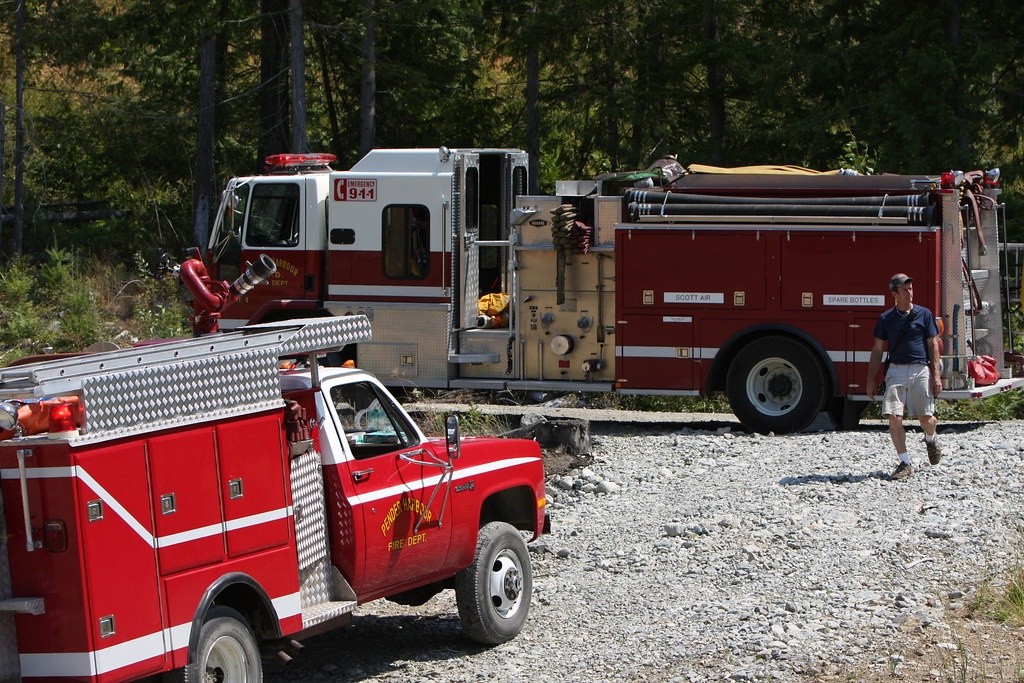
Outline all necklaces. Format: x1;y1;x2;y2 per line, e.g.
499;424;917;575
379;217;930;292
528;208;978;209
896;305;910;317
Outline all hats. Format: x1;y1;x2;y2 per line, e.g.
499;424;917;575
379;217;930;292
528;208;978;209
888;273;912;291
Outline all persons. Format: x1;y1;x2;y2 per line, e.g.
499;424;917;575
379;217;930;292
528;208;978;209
866;274;943;480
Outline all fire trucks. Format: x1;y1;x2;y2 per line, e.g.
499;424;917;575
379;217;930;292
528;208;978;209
206;147;1024;435
0;248;551;683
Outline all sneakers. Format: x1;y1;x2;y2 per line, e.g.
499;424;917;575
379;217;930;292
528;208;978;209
892;461;912;480
924;430;941;465
